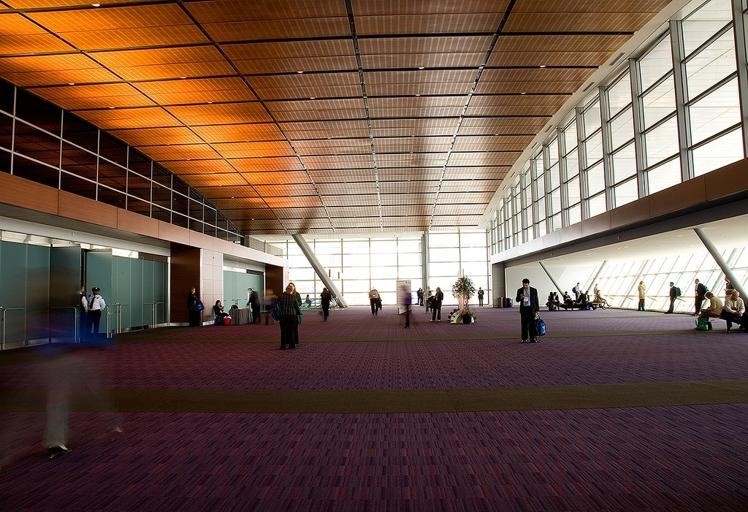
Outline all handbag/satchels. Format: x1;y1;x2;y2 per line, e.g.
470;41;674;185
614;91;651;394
719;307;741;324
271;300;282;321
695;317;708;331
534;314;546;336
194;303;204;311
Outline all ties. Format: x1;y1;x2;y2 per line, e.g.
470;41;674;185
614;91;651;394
90;295;95;309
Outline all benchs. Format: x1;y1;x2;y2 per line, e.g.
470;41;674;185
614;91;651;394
550;298;605;310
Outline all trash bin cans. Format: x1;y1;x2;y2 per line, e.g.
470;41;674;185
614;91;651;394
497;296;513;308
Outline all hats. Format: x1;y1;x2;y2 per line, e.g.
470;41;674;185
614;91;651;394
92;287;100;291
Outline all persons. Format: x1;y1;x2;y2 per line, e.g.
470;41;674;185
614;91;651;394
188;286;204;327
28;291;115;462
546;283;609;311
211;282;444;351
637;280;646;311
477;287;485;307
516;278;541;344
664;276;747;333
71;283;90;343
88;286;108;336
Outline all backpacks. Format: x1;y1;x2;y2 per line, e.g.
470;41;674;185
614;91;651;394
676;286;681;297
